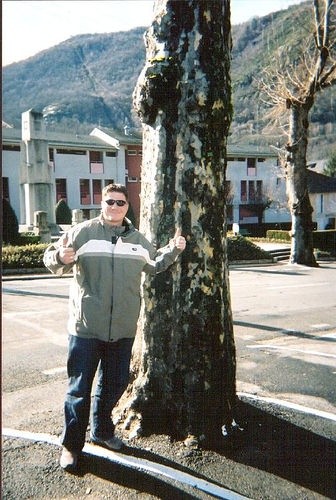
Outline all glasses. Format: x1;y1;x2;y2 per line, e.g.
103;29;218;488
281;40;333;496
105;199;127;206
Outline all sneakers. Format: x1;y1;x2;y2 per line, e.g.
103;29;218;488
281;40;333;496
90;432;122;450
60;445;78;470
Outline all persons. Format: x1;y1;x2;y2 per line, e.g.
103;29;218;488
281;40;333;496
42;182;186;472
